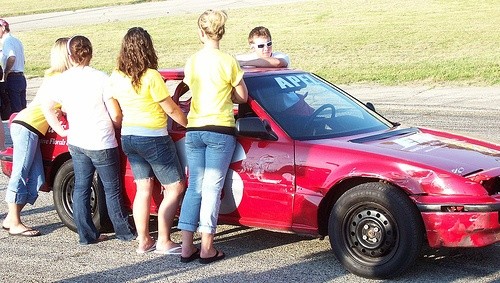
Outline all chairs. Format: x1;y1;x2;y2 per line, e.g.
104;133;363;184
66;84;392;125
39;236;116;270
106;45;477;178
235;96;258;119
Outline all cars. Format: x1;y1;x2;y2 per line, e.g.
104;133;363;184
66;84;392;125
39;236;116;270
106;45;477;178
1;64;496;276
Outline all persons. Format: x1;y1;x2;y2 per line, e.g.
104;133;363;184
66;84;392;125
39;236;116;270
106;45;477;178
42;36;137;244
112;27;188;254
0;19;27;113
234;26;300;112
176;10;248;264
3;37;73;237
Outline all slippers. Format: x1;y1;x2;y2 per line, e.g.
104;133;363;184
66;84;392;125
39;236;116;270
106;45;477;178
136;239;158;254
180;247;201;263
8;228;41;237
2;226;10;231
153;243;183;255
198;250;225;264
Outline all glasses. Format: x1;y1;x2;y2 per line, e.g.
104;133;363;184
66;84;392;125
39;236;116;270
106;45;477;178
252;41;272;48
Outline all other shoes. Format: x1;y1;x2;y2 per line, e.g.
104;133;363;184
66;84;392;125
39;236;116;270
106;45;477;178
95;235;109;242
